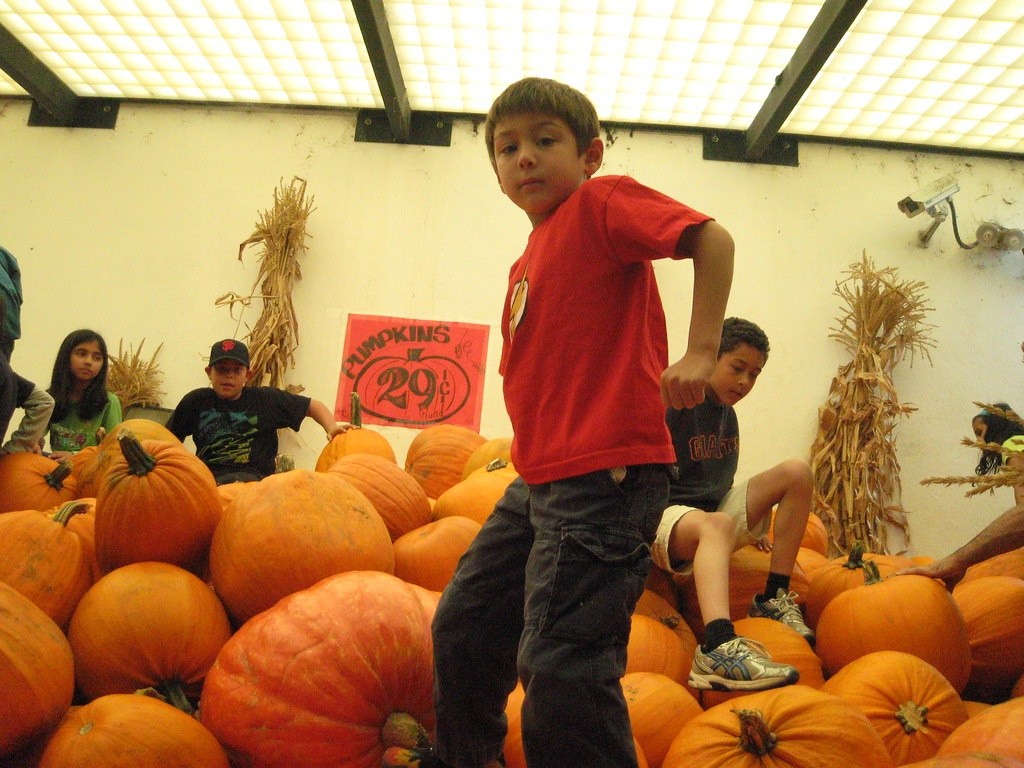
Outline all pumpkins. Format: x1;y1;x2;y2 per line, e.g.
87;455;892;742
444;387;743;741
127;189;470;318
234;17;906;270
2;390;1023;768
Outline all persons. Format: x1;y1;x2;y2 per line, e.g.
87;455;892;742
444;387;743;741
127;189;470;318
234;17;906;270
423;66;739;768
890;495;1023;599
644;316;818;696
168;337;370;495
24;328;127;464
0;246;59;470
970;400;1023;512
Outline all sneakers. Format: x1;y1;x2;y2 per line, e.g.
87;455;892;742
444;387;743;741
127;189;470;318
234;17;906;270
687;641;799;692
747;591;816;637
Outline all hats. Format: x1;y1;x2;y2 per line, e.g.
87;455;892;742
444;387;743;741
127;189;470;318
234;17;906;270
210;339;250;366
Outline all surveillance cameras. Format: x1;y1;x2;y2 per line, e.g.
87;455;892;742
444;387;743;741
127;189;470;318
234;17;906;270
897;173;960;219
